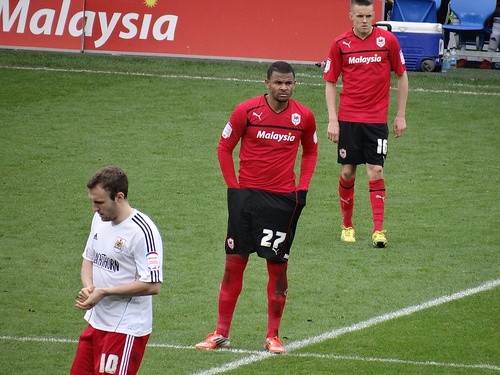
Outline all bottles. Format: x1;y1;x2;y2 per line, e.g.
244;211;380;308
443;48;458;73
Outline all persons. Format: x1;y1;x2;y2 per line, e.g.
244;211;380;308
195;61;317;355
457;0;500;68
324;0;409;247
69;167;163;375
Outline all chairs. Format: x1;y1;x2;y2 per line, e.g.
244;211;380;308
390;0;497;50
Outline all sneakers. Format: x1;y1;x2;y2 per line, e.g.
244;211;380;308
195;329;231;350
263;335;285;353
457;59;467;67
371;229;388;248
480;60;492;69
340;224;357;242
